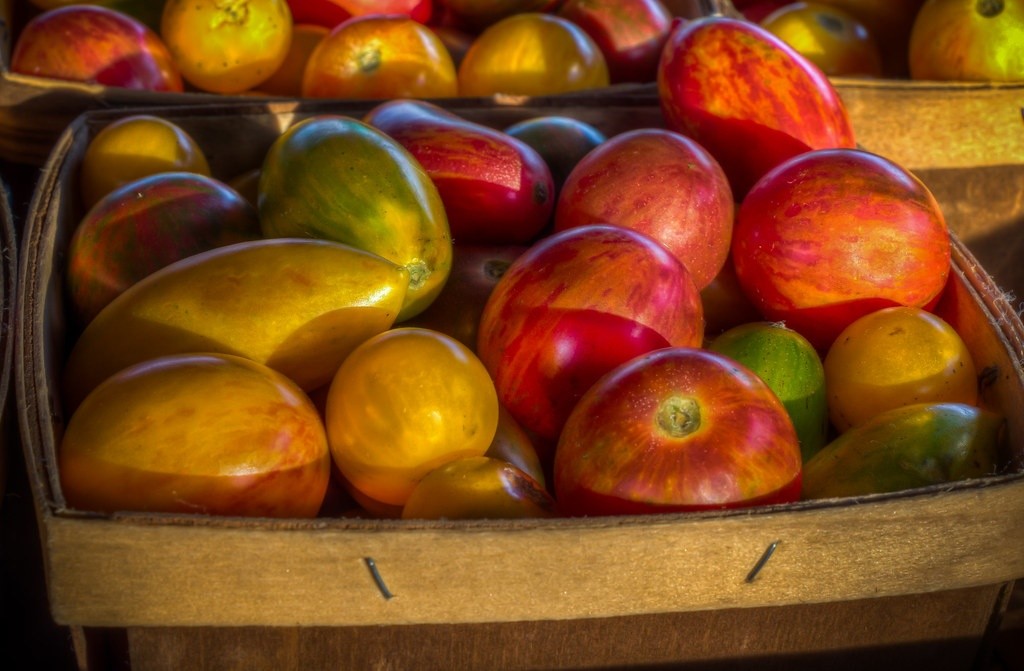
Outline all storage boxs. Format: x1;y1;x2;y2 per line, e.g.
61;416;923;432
0;0;1024;671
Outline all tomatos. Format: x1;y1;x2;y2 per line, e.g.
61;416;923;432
11;0;1024;522
